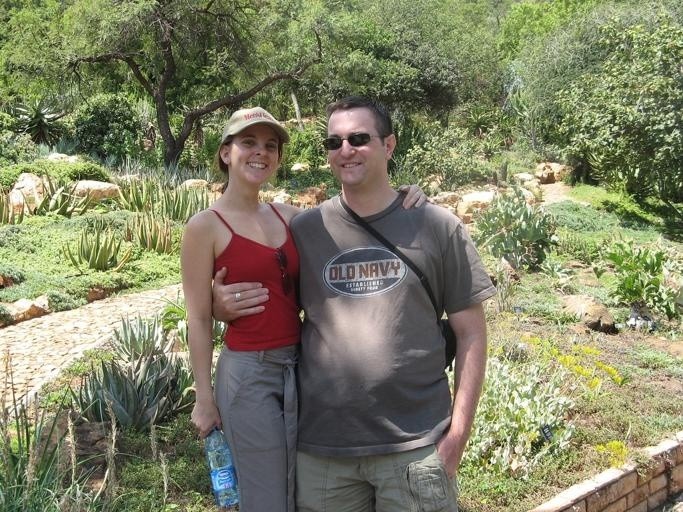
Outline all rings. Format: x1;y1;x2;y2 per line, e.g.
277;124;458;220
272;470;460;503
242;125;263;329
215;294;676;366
235;292;240;302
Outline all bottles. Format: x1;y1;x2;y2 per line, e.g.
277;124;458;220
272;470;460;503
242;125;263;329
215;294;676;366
204;428;240;508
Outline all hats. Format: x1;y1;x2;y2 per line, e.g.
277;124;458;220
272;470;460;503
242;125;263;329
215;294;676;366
219;107;291;146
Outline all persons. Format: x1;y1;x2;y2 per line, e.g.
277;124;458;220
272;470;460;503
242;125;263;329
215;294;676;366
212;93;497;512
179;107;435;512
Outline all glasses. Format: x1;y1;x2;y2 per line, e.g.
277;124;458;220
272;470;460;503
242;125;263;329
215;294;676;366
275;246;295;299
322;132;389;150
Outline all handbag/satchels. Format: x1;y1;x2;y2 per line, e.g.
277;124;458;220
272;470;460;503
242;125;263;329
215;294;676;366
436;316;456;371
